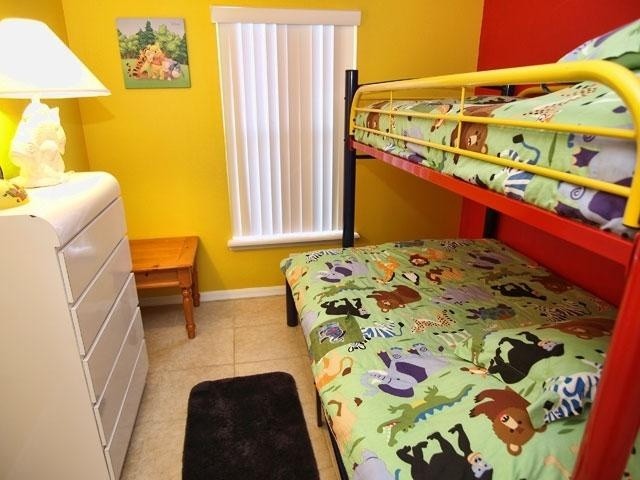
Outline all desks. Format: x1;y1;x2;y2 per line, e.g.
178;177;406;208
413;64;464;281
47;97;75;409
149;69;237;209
130;235;200;340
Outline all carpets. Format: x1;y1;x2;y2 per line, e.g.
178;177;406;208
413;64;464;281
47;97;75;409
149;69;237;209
181;371;321;480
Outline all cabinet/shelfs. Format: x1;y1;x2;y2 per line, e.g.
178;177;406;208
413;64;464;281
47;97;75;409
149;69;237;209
1;170;150;480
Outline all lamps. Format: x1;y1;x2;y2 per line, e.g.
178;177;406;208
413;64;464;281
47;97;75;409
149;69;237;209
0;17;112;187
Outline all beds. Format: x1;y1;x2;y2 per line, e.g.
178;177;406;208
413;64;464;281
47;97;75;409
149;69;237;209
284;69;640;480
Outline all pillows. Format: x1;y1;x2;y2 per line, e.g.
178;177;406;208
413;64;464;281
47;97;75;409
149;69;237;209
454;311;617;424
553;17;640;71
